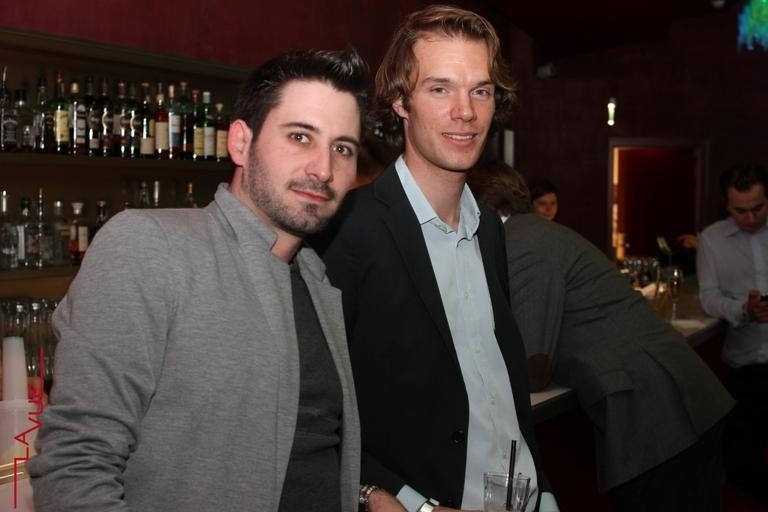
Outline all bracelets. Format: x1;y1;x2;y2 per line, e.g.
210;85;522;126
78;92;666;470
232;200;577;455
357;482;380;507
417;498;440;512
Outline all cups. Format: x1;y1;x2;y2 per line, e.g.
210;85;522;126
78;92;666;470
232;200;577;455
482;472;530;511
668;269;684;302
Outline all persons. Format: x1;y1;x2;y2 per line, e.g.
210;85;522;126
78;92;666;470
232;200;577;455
315;3;561;512
530;179;561;223
693;167;767;404
676;231;696;253
471;160;744;512
27;39;410;511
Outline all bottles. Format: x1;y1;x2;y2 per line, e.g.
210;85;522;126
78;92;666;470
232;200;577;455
0;296;62;379
618;254;662;288
0;177;201;270
1;67;232;165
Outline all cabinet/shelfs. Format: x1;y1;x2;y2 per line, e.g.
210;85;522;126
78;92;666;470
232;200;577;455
0;26;254;395
